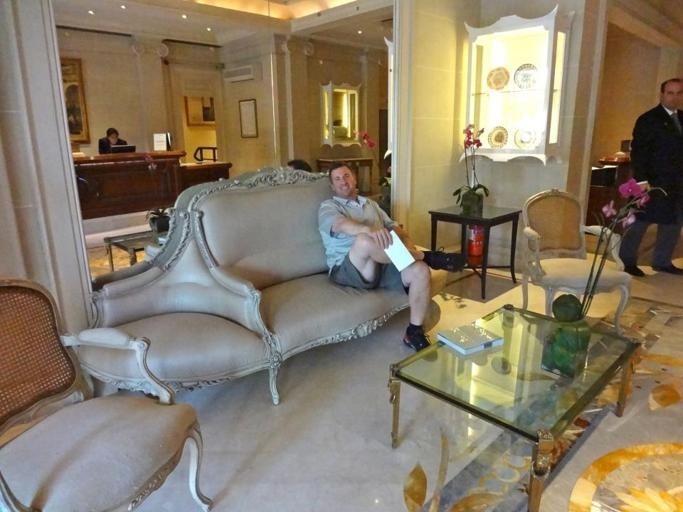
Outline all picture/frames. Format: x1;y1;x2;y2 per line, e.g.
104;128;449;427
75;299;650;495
183;94;216;128
57;56;90;146
237;99;259;140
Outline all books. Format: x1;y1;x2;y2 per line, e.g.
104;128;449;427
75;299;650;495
437;325;504;354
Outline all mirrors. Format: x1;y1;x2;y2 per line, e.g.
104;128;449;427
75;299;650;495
39;0;395;293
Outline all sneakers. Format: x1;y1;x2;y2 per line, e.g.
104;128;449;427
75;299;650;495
426;247;466;273
402;327;436;361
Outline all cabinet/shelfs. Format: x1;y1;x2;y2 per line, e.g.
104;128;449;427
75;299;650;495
317;79;362;150
457;4;575;166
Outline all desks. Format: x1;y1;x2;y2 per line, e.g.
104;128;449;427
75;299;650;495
585;150;634;236
426;204;522;301
103;230;169;274
316;156;373;198
71;152;233;221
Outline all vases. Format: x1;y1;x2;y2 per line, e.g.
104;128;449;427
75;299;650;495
537;320;591;383
381;185;390;208
460;192;484;217
148;216;169;233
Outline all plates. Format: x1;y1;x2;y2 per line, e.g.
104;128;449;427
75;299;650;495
512;63;539;90
486;126;508;149
486;67;509;90
513;128;535;150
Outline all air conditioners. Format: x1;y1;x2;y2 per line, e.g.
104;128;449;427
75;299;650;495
221;63;262;85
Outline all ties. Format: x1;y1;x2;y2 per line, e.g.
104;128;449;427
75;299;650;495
669;112;683;137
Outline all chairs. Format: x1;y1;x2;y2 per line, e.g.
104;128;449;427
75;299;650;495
0;277;212;512
521;187;633;338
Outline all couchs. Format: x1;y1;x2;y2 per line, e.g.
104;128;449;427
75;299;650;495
75;170;449;407
88;163;275;291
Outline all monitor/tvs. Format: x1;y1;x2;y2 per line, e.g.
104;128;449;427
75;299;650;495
165;131;172;152
110;145;136;154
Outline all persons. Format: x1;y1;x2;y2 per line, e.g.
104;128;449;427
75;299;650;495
617;78;683;277
318;163;466;361
98;128;127;154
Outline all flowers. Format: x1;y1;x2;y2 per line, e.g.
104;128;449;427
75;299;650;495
356;130;390;193
452;123;492;208
139;152;171;222
551;176;668;322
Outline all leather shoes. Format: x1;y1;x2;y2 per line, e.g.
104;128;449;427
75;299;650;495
623;261;644;277
653;262;682;275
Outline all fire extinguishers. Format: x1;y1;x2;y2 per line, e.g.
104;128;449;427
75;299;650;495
467;225;483;257
